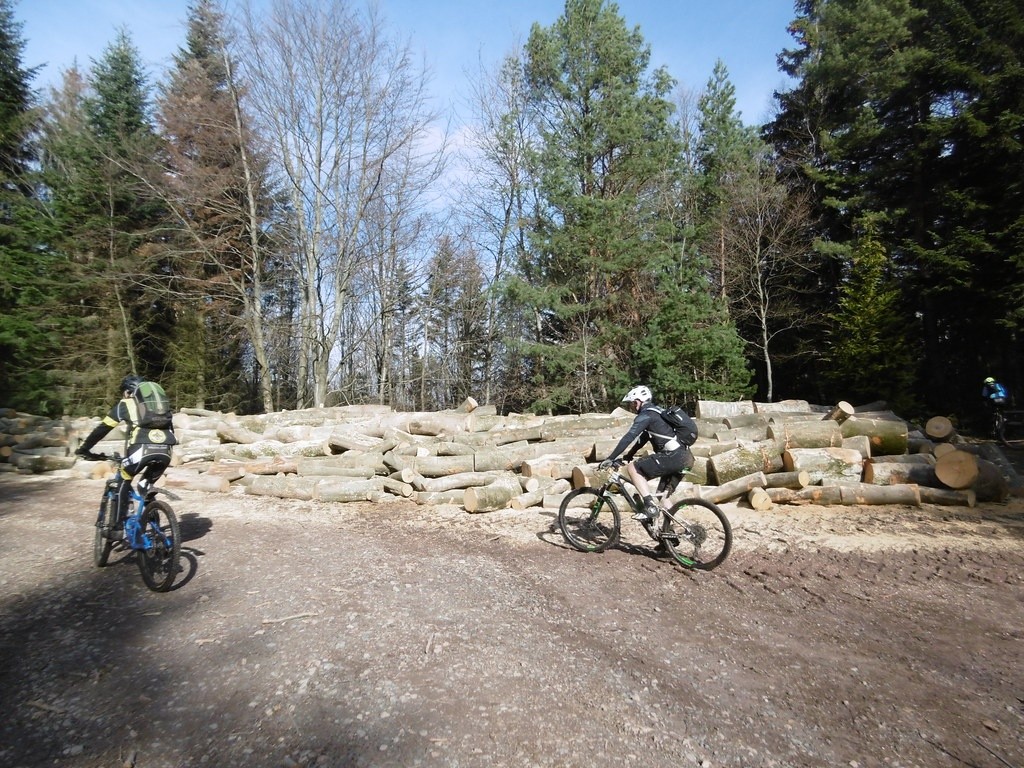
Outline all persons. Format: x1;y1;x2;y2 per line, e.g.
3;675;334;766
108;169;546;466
74;374;180;541
982;376;1009;435
597;385;695;551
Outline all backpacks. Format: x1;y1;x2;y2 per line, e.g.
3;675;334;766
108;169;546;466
637;405;699;448
133;380;173;430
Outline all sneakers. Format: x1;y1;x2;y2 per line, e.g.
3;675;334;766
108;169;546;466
99;527;124;539
632;503;660;520
655;537;679;551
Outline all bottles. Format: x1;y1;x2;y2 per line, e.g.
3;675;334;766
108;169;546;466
633;492;644;509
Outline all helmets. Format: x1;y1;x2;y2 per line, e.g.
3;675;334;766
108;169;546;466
120;373;144;392
621;385;652;403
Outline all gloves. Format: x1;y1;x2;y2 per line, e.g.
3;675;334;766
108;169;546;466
75;445;90;459
599;458;612;470
622;454;632;462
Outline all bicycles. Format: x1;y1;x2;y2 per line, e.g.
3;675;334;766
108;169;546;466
556;455;734;573
87;452;182;594
983;398;1024;452
